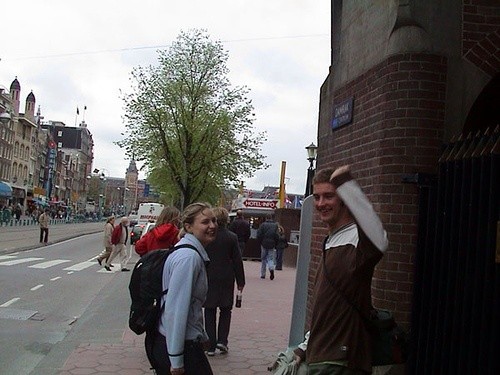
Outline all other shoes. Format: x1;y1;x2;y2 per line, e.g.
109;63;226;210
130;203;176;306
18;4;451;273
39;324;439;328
97;257;130;272
260;269;275;280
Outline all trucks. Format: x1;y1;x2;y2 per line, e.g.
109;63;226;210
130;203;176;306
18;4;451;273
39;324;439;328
136;203;165;226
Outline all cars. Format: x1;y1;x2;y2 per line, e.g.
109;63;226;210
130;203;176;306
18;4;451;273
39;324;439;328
141;222;156;239
128;214;138;226
130;224;146;244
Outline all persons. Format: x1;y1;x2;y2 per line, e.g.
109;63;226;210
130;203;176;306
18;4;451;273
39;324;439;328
39;208;49;246
202;208;246;356
0;203;125;226
135;206;181;258
230;210;287;280
144;203;215;375
294;164;389;375
95;216;131;271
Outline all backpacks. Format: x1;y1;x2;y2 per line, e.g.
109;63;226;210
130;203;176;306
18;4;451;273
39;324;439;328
129;244;205;335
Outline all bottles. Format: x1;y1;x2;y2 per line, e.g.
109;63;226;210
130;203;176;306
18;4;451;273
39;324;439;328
236;290;242;308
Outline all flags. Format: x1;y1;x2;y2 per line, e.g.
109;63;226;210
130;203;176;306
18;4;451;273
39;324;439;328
77;108;79;114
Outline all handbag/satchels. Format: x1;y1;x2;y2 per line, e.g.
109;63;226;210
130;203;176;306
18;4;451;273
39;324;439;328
366;307;409;367
262;239;274;249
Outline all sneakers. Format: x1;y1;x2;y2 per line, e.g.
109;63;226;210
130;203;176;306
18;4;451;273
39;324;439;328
208;352;215;356
217;343;228;352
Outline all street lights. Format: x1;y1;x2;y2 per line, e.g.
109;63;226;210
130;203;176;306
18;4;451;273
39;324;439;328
304;143;317;198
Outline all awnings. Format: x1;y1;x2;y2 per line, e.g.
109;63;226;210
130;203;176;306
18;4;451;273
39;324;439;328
0;181;26;199
